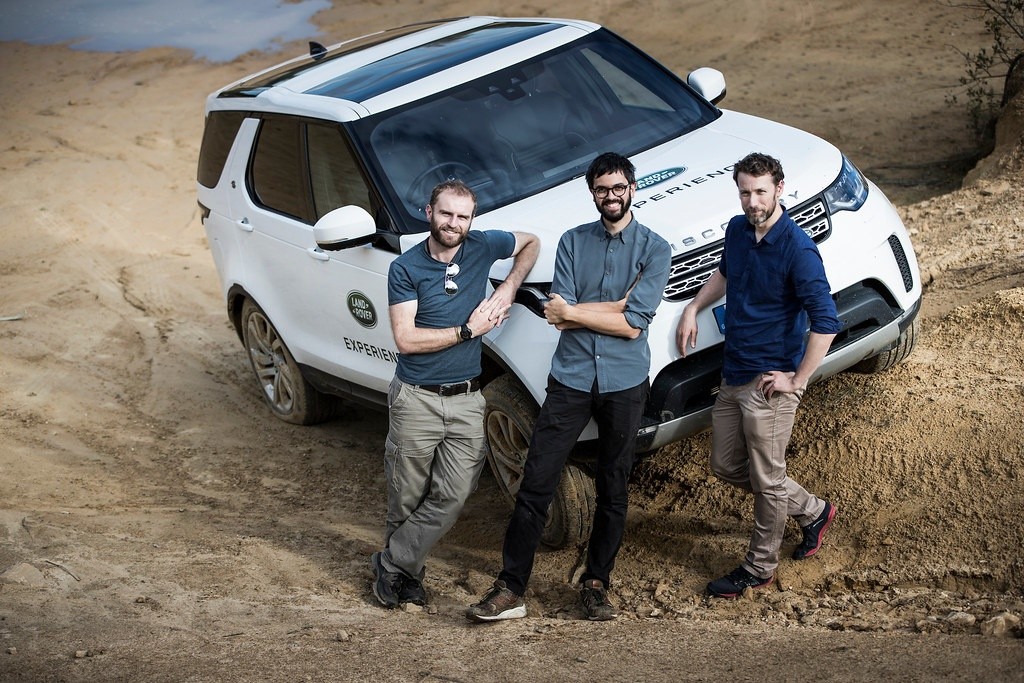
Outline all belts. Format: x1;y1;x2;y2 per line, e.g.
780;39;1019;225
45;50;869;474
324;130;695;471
410;377;480;396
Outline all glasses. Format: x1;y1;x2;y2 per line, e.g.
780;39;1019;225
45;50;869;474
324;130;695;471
445;262;460;295
593;185;630;198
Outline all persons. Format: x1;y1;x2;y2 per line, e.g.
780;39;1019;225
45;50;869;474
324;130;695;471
371;181;541;609
466;153;672;622
676;153;839;597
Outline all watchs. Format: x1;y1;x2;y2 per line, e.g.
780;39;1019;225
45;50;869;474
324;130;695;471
460;324;472;341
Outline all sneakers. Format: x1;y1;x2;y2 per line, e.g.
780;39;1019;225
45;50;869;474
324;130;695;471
706;563;774;597
466;580;527;621
792;502;835;560
580;579;617;620
371;552;426;607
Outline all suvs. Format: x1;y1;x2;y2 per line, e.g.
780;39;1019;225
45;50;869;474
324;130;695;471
195;15;925;552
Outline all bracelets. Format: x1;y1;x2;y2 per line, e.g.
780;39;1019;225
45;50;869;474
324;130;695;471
454;326;460;344
